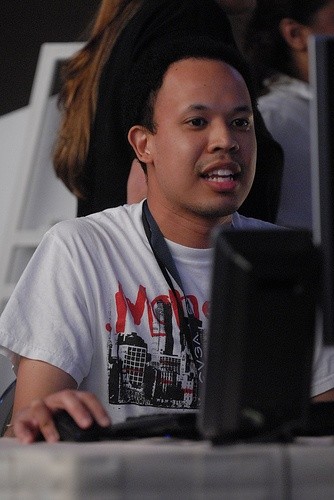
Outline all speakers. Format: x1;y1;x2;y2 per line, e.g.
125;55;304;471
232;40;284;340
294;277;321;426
199;225;325;443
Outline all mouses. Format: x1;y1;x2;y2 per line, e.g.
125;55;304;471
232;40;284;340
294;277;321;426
35;408;114;442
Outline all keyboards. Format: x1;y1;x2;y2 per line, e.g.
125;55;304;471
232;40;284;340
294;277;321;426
106;412;205;439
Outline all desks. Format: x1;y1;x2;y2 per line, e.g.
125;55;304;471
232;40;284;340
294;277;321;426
0;434;333;500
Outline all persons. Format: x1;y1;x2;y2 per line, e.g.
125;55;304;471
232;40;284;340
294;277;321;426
241;0;334;236
52;0;283;225
0;34;334;444
